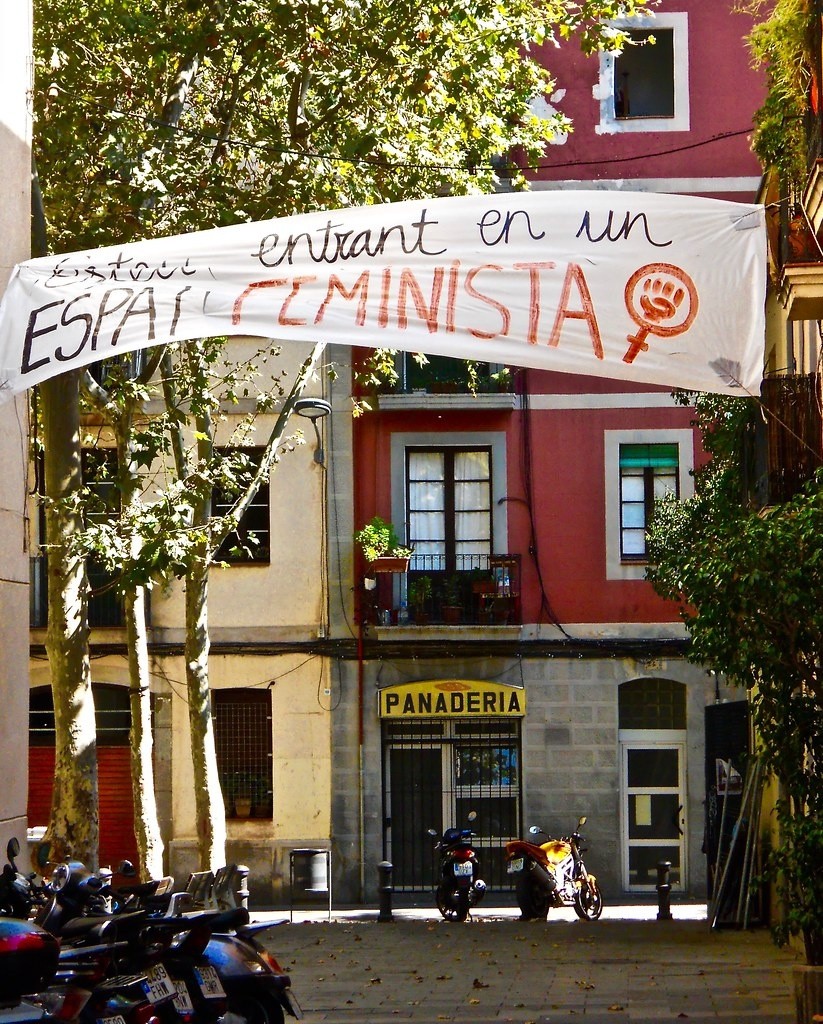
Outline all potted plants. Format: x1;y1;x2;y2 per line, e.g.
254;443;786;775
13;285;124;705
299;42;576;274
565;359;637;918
359;350;516;395
357;517;413;573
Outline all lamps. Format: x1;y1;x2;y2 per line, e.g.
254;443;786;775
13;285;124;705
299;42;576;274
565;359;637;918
295;396;332;462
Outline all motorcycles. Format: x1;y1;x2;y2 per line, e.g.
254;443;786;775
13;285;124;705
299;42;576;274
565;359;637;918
1;836;305;1024
426;812;487;922
503;815;604;919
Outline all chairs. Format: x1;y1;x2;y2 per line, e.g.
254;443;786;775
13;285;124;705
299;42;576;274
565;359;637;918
139;863;237;921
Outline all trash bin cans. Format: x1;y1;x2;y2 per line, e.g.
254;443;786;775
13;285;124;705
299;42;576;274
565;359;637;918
292;849;328;894
